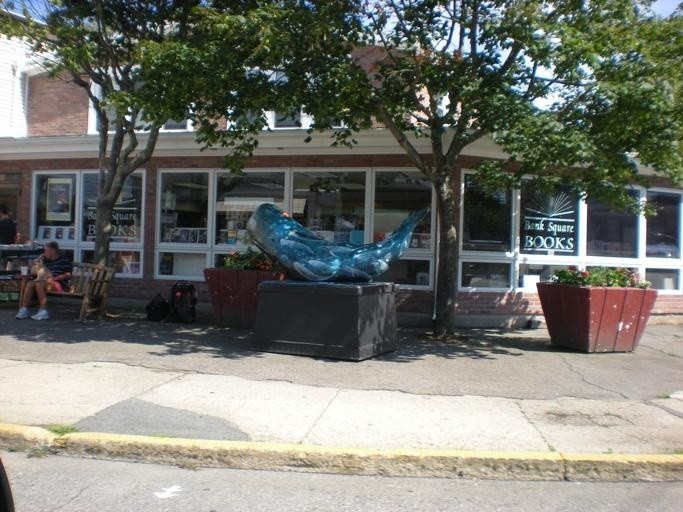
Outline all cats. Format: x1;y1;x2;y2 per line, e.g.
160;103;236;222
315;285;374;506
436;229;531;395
32;258;53;282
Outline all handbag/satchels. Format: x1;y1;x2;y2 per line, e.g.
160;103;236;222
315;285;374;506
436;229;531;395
146;293;167;322
168;303;189;324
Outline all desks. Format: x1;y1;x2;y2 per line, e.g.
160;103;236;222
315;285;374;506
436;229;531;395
0;244;44;308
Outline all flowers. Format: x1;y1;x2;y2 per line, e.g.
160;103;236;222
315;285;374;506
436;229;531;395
225;248;276;271
554;266;650;287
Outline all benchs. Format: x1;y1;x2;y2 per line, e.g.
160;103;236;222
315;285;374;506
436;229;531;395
0;270;24;308
18;261;115;324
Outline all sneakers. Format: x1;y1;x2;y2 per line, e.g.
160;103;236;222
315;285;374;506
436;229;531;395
30;309;49;321
15;308;29;319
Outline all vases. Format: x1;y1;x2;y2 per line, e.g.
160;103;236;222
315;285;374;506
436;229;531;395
537;281;657;353
203;269;289;328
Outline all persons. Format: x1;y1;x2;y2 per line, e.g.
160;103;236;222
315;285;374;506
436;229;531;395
17;241;72;321
0;207;18;288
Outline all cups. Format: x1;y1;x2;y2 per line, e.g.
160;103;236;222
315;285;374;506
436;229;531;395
20;266;27;275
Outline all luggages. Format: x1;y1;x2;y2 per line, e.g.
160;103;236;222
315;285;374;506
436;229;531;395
170;280;196;314
